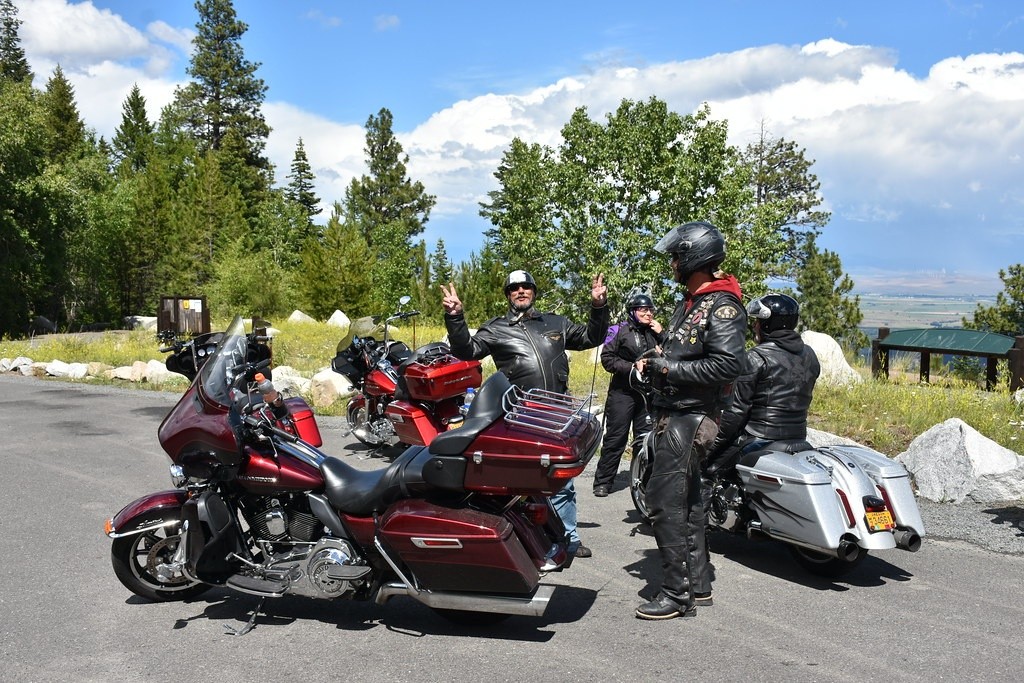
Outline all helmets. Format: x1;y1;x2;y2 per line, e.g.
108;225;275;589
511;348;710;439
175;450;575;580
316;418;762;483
503;270;536;291
665;222;726;280
745;293;799;321
626;294;653;308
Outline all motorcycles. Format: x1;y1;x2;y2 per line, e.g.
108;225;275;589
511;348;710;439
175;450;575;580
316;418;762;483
330;295;483;448
102;313;606;639
629;350;926;565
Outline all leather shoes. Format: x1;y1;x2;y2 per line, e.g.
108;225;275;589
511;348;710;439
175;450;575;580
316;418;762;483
563;539;591;558
635;586;713;619
593;480;613;497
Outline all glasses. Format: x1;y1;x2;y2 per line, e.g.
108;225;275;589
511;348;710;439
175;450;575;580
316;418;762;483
507;282;535;291
634;306;655;313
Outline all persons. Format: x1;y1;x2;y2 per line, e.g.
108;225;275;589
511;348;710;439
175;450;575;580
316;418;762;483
635;222;747;620
440;269;609;558
593;295;667;497
701;293;820;563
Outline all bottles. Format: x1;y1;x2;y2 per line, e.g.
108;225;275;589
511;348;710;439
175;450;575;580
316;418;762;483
255;373;288;419
464;388;475;415
